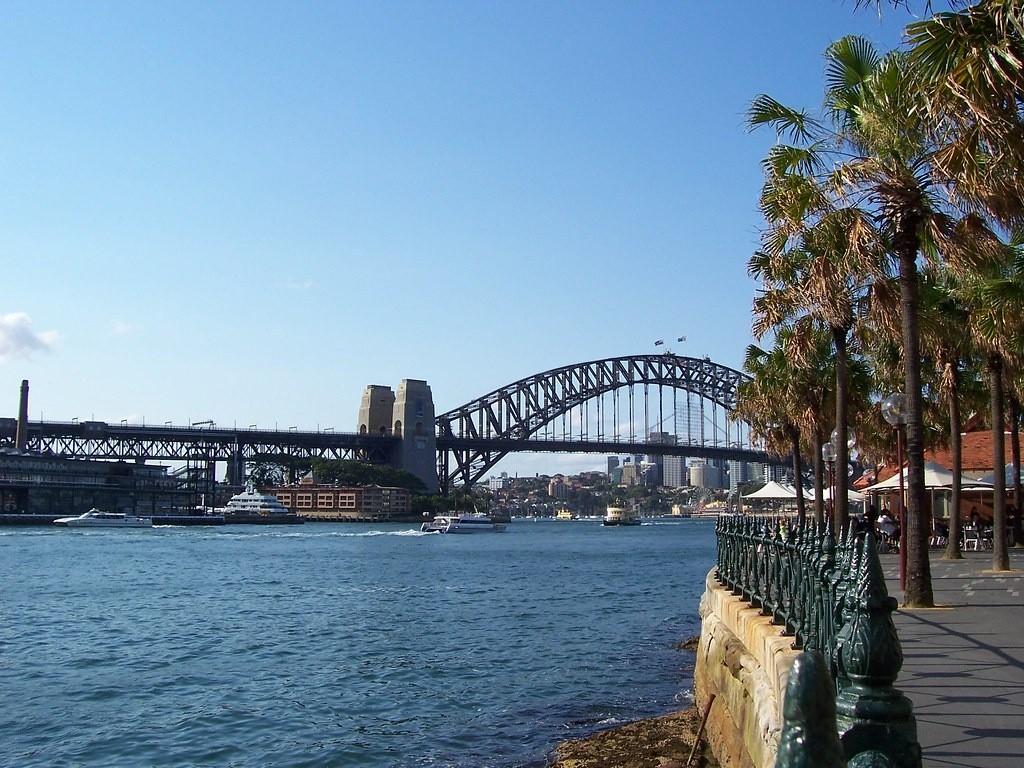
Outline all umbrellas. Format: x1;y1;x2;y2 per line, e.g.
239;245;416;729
742;459;1024;530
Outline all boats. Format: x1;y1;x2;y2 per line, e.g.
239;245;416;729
422;514;507;535
52;507;154;528
222;475;289;514
602;499;641;526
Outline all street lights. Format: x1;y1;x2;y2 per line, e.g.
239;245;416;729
823;425;861;535
881;393;908;591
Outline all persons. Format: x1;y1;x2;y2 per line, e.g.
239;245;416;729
760;504;1018;542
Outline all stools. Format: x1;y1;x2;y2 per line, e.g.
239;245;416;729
964;538;980;551
931;535;945;546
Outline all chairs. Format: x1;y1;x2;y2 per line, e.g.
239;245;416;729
873;521;894;552
